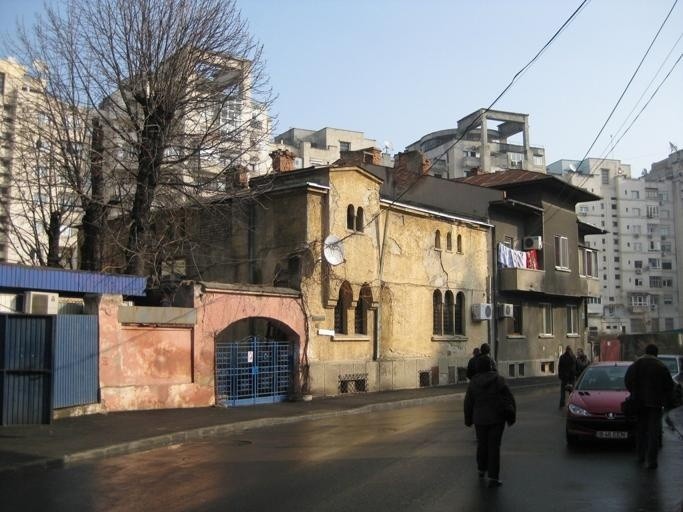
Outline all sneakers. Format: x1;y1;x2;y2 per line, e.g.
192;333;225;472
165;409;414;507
478;470;502;486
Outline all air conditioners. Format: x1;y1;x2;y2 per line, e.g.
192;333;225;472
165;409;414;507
21;291;59;316
470;301;514;321
523;235;542;250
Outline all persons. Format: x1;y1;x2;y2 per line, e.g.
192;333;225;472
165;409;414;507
461;355;517;488
467;347;483;382
470;342;498;375
555;344;577;408
624;343;675;470
574;348;591;380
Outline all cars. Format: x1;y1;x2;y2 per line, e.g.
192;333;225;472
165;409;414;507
563;360;663;453
